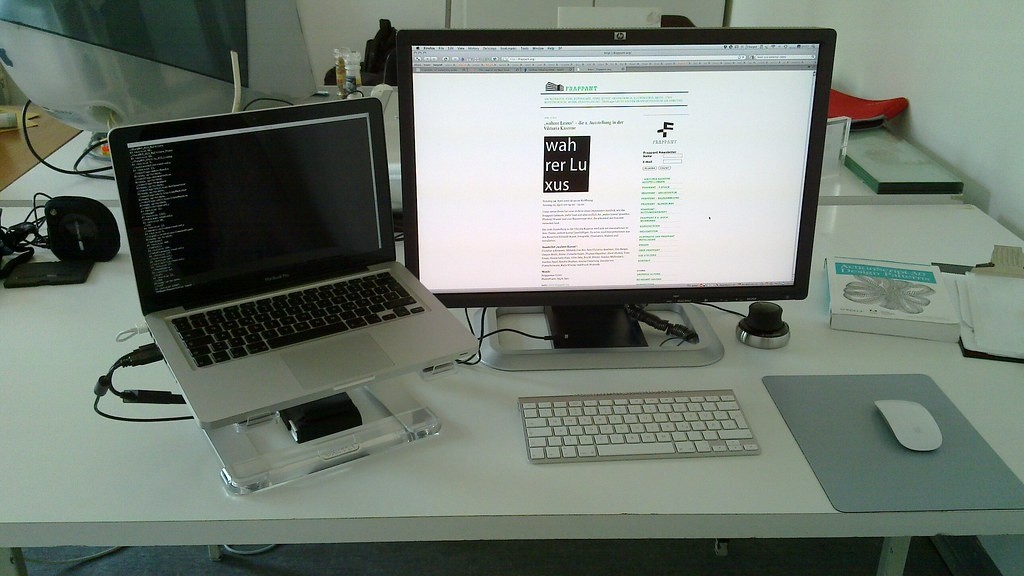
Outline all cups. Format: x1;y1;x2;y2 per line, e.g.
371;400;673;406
332;47;361;99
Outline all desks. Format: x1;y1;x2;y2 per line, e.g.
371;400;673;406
0;127;1024;576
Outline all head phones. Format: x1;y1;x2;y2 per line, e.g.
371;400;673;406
0;192;121;263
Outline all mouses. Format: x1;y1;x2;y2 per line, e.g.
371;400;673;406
875;398;942;451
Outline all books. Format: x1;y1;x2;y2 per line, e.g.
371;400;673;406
839;126;965;194
823;256;961;343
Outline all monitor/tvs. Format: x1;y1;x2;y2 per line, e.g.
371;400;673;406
0;0;316;162
395;29;837;368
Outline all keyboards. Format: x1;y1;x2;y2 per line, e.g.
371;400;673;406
518;389;761;464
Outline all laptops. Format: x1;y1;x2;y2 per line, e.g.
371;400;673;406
106;97;479;428
